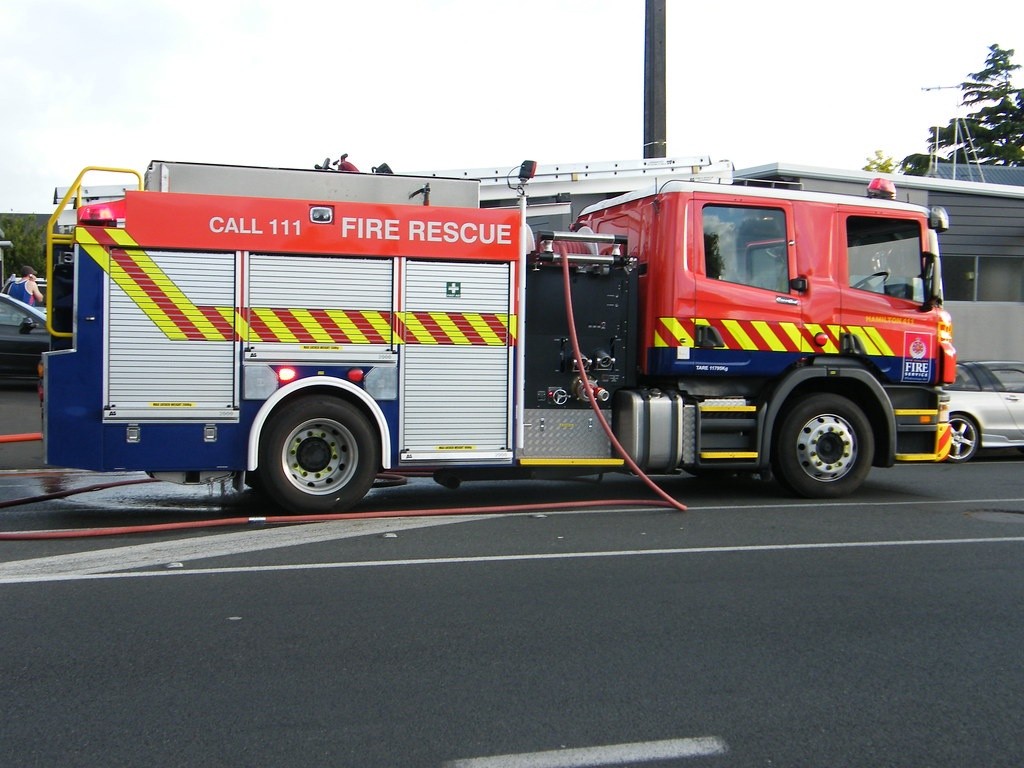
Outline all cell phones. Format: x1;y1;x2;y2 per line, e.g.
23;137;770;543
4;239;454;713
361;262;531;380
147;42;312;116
29;273;32;278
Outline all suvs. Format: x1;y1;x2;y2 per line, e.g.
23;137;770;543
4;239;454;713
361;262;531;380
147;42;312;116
3;273;48;316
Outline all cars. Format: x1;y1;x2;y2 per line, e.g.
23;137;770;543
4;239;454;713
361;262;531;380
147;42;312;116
0;293;48;381
943;360;1023;464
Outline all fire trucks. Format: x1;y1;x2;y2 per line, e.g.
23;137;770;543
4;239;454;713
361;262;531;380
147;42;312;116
35;152;959;519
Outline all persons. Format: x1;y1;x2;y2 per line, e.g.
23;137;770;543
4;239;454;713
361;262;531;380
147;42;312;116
7;266;43;306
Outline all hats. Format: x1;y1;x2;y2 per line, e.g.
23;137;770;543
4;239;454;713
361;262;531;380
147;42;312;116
21;266;37;276
